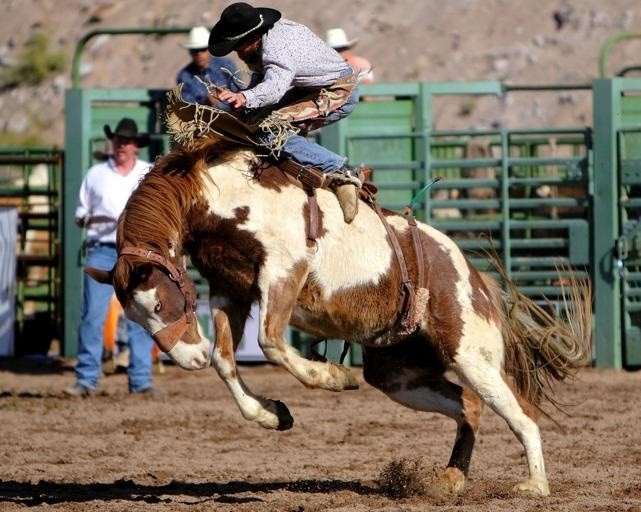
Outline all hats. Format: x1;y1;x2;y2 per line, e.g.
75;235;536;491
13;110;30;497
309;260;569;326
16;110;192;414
104;118;151;147
179;27;211;49
325;29;358;50
208;3;281;57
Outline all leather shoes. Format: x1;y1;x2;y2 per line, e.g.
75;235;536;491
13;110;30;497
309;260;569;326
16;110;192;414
64;384;88;395
330;166;358;223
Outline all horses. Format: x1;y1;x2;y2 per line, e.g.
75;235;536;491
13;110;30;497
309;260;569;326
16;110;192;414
459;143;592;299
83;136;594;497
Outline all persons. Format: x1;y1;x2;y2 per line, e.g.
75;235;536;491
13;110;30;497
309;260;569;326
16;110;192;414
325;28;376;103
61;117;170;400
174;25;244;115
206;2;361;225
